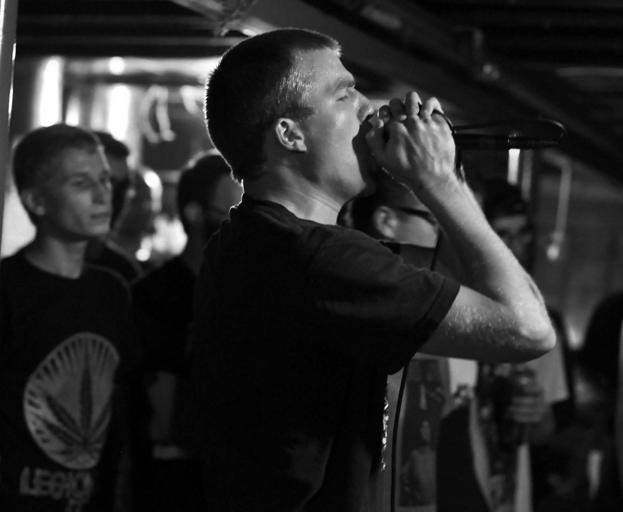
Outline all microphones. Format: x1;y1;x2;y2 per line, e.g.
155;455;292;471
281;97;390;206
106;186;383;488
451;119;565;150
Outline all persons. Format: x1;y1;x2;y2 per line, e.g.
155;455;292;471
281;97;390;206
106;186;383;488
201;30;558;510
349;176;623;510
1;123;243;510
